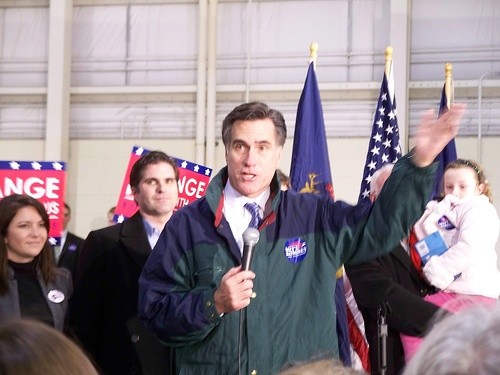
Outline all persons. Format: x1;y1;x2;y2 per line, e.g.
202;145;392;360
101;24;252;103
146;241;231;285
74;150;182;375
342;163;458;375
137;100;466;375
0;194;74;335
278;174;291;192
50;202;85;272
398;159;500;367
107;205;118;227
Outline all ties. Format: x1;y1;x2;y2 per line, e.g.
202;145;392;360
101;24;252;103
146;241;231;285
409;227;423;278
243;202;262;230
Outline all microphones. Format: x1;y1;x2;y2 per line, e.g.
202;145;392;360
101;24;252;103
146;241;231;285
241;227;259;271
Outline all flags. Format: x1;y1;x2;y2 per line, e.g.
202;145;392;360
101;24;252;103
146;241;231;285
288;43;351;368
423;62;457;200
338;45;408;375
